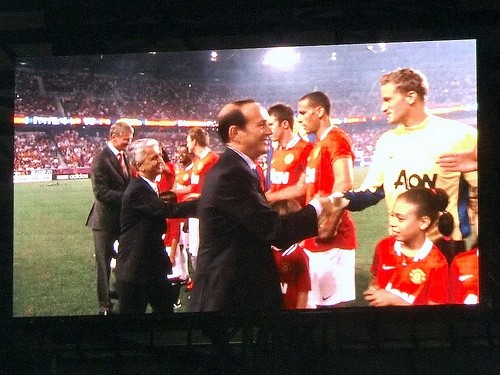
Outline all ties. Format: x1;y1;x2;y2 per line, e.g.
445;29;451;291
154;183;160;199
255;165;265;192
118;153;126;174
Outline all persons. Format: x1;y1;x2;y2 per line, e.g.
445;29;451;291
115;137;200;313
264;103;313;210
296;91;358;309
85;121;134;315
327;68;478;267
435;146;478;172
133;126;219;293
361;187;454;306
184;99;334;311
269;199;311;309
13;69;394;174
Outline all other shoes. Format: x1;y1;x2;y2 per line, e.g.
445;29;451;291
173;300;181;308
99;310;113;315
